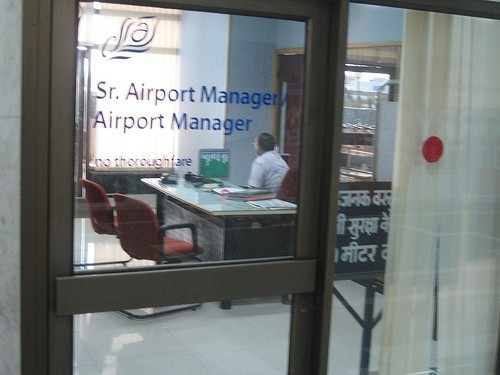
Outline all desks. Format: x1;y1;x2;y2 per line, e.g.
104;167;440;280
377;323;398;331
141;176;299;310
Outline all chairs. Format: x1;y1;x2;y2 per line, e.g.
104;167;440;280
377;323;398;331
71;178;136;279
105;194;206;319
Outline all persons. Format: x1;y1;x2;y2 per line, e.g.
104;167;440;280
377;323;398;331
247;132;291;196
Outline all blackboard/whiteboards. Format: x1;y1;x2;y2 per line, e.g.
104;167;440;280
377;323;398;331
198;148;230;180
331;181;395;280
